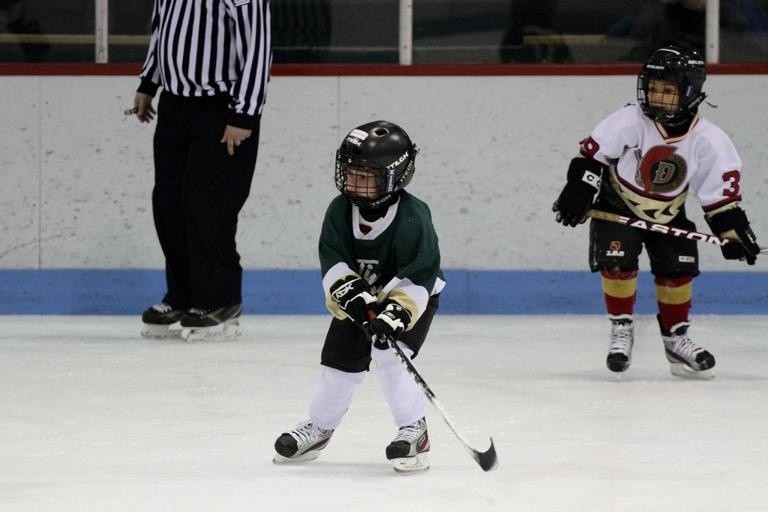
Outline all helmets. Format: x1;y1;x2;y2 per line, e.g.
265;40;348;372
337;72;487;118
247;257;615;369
334;119;418;209
635;46;707;125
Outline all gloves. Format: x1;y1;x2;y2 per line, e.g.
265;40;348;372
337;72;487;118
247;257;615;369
551;155;607;229
704;206;760;266
330;274;413;350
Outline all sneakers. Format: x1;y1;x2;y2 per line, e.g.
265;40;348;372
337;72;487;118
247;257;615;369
275;422;334;457
386;417;430;459
607;313;633;373
181;305;241;327
661;320;716;371
142;303;181;325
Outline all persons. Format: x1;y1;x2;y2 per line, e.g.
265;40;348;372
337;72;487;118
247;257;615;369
271;120;445;475
133;0;274;341
551;42;759;382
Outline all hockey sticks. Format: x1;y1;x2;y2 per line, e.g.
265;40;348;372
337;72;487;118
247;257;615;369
366;309;497;471
